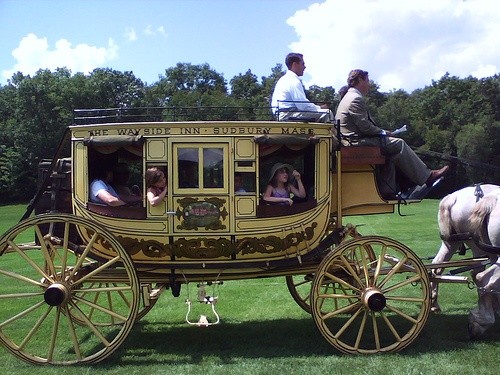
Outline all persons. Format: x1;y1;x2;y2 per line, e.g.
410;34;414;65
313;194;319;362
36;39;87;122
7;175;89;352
271;53;334;123
335;69;450;199
234;174;246;192
263;162;306;206
89;160;143;207
146;168;167;207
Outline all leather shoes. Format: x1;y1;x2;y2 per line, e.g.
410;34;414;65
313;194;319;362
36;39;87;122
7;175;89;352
399;179;409;192
425;165;449;189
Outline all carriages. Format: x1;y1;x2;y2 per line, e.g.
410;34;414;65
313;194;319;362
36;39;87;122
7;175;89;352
0;102;499;366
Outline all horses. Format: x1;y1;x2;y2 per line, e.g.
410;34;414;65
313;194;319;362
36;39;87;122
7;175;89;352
430;183;500;338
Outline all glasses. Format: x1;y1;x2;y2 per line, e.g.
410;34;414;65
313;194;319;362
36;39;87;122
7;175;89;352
277;170;290;174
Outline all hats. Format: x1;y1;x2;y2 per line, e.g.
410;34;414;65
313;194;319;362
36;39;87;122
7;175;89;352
268;162;294;184
